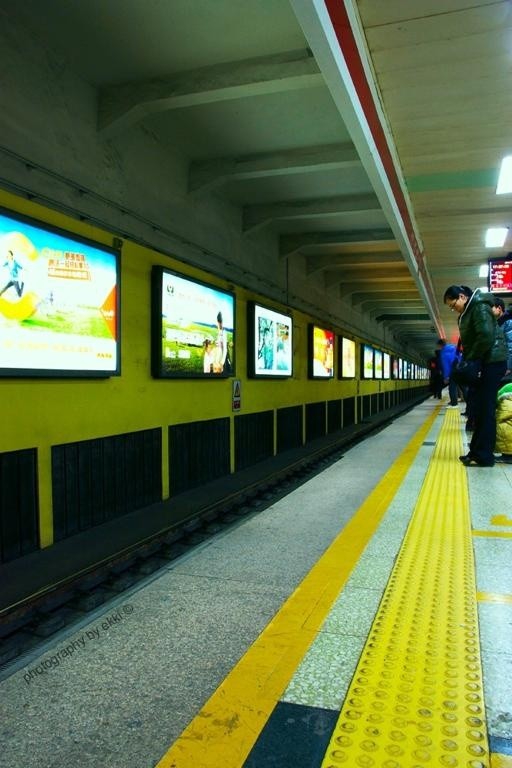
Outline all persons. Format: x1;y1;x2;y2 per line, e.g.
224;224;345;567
259;329;275;370
0;246;26;298
217;312;232;372
201;337;214;374
424;283;512;469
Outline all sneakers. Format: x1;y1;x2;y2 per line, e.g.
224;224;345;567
460;453;494;467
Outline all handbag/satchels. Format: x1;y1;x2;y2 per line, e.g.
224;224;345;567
449;357;483;387
